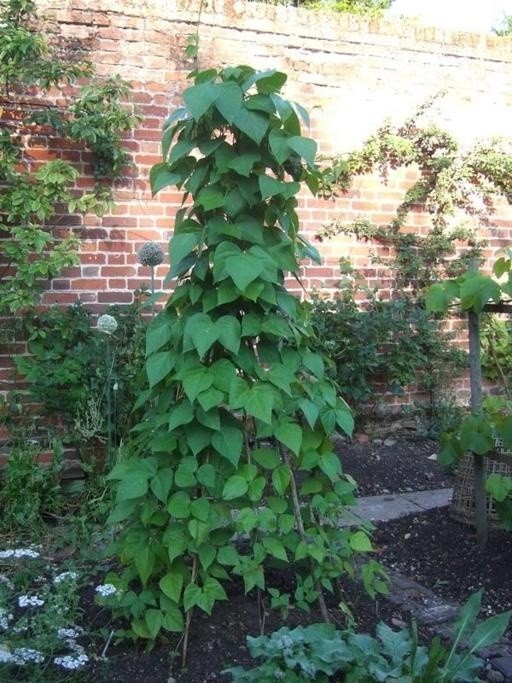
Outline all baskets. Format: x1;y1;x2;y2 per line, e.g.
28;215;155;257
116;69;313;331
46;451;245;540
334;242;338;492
450;433;512;532
